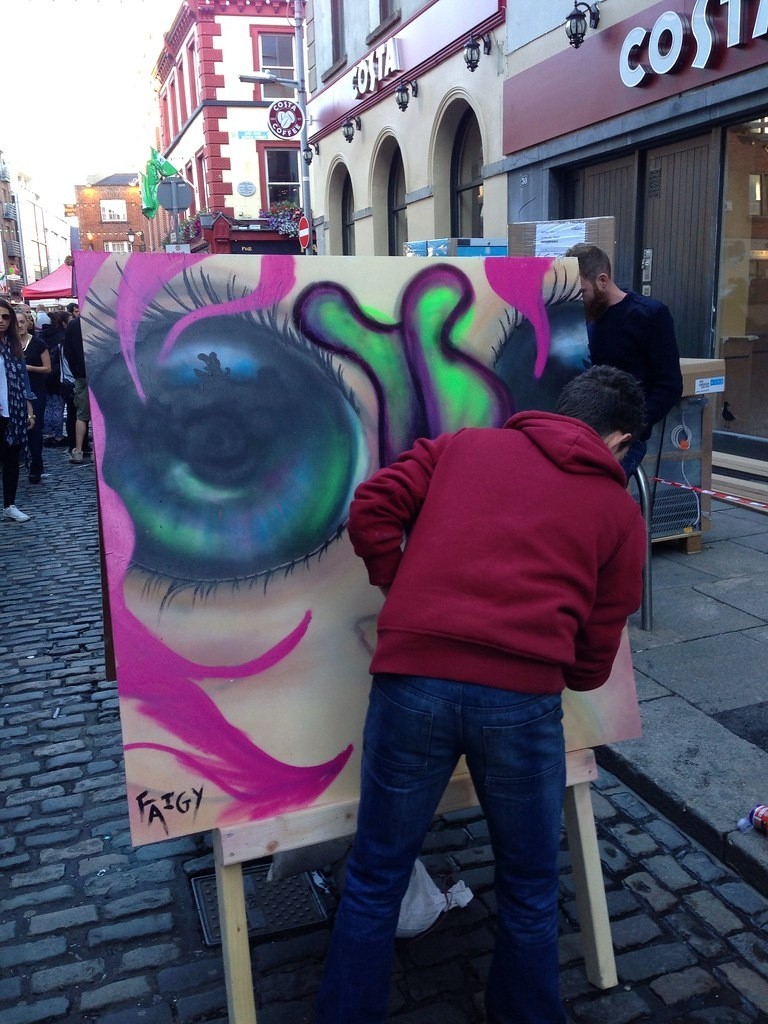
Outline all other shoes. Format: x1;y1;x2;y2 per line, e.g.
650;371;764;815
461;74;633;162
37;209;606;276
44;437;52;449
61;445;72;453
52;436;68;446
82;443;93;452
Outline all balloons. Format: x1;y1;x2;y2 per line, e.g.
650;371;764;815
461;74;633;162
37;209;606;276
8;257;20;275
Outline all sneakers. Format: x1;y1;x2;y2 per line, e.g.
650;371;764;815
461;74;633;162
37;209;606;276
90;450;95;463
69;448;83;463
3;505;31;523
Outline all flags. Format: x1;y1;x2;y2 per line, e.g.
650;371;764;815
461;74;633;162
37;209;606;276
147;147;178;207
142;175;160;219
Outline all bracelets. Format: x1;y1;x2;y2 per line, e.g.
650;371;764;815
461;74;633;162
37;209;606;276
28;415;36;419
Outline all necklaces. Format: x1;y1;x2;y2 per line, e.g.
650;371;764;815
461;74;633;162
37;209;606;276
23;339;29;351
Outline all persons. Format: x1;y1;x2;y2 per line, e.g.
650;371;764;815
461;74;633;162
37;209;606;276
316;364;650;1024
565;243;683;487
0;298;92;523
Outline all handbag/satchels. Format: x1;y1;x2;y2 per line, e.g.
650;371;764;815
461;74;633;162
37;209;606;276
60;380;75;404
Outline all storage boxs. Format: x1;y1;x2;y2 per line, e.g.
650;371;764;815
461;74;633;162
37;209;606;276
679;357;726;396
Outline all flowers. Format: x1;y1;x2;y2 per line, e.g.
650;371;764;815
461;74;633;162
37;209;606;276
179;207;207;244
261;201;305;238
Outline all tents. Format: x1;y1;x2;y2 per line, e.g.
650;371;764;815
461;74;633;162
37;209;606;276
20;263;73;303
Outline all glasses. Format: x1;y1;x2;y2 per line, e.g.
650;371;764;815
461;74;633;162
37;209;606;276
0;314;12;320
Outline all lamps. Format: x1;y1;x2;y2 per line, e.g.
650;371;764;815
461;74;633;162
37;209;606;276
565;1;601;50
464;33;491;72
394;78;418;112
303;143;319;166
342;115;361;142
238;70;299;88
